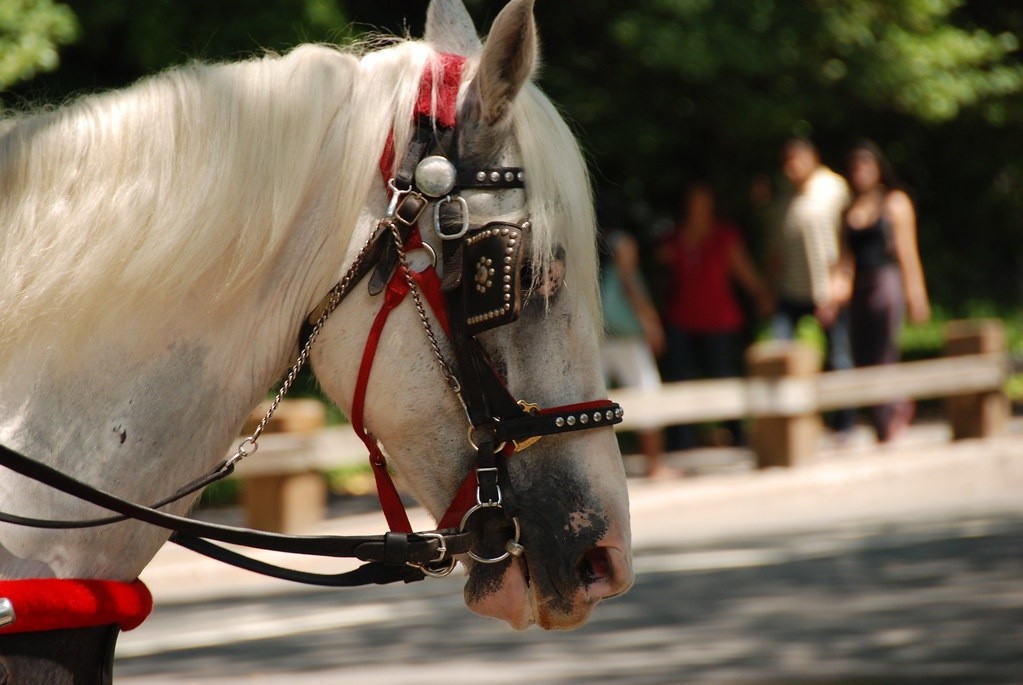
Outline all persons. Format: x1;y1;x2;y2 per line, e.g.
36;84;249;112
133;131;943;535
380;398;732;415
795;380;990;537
601;224;666;455
771;137;930;442
664;184;774;446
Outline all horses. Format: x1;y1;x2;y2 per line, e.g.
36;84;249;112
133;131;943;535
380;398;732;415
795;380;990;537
0;0;634;685
